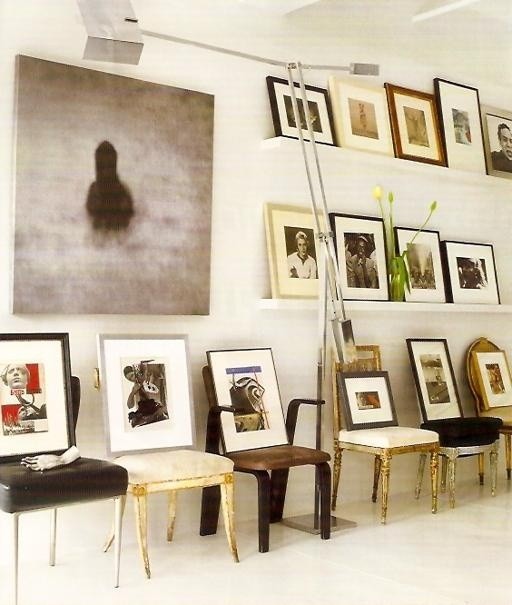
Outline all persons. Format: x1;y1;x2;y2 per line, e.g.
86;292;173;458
287;231;316;279
19;445;81;471
123;360;165;410
0;363;31;390
491;123;512;173
346;235;378;289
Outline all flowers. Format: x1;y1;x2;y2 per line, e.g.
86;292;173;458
371;184;437;257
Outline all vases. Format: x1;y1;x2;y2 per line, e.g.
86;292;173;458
389;253;406;304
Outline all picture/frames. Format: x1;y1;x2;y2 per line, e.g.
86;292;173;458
260;202;331;303
205;347;295;457
443;240;502;308
472;348;512;415
0;331;80;466
265;73;511;181
96;330;199;459
393;222;442;302
337;369;403;434
325;212;391;306
406;336;465;422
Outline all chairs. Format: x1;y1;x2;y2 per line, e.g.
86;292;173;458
100;372;245;585
197;362;335;558
404;340;512;517
0;373;131;605
329;343;441;528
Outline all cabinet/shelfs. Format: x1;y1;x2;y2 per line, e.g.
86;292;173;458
258;133;511;315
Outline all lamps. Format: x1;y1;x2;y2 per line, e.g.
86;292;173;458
75;0;392;538
